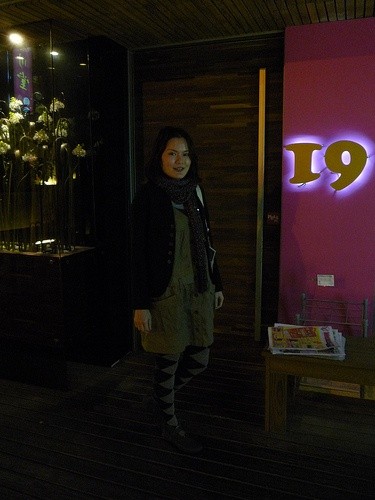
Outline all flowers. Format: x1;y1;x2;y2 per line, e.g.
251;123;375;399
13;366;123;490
0;97;87;186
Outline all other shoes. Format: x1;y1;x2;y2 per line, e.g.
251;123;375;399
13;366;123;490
144;395;157;414
161;424;201;452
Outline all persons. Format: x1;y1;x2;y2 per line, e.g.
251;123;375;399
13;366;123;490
129;124;226;454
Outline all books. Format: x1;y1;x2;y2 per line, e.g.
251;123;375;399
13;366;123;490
267;322;347;361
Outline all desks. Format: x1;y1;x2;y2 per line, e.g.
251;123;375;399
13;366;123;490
265;336;375;434
